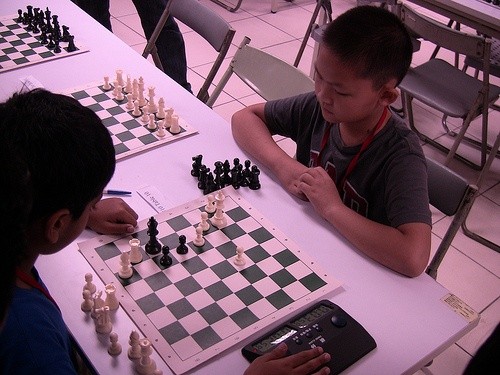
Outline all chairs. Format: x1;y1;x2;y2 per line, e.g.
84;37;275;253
212;0;242;12
461;135;500;254
294;0;343;80
393;3;499;167
410;157;478;278
390;15;455;114
442;33;500;156
140;0;235;102
205;36;316;115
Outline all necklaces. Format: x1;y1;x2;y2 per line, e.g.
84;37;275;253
317;108;388;193
14;269;60;310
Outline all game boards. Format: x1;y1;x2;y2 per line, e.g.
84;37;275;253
77;185;344;372
47;69;199;162
0;6;90;74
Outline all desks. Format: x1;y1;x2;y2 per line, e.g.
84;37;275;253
412;0;499;35
0;0;480;375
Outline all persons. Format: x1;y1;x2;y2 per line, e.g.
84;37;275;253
76;0;193;94
232;5;433;278
0;88;331;375
